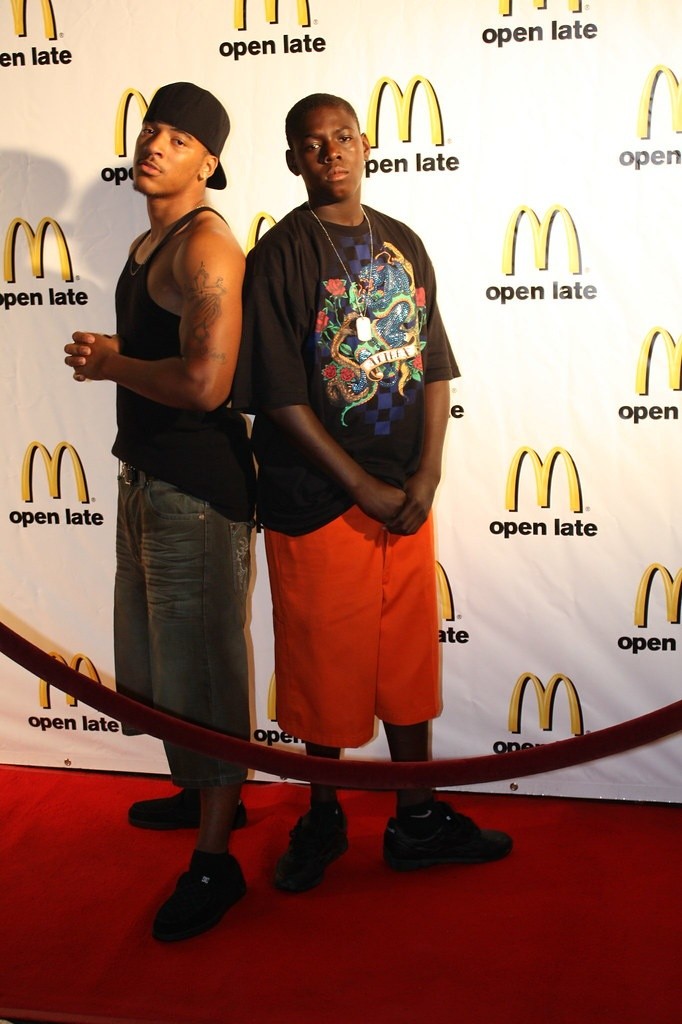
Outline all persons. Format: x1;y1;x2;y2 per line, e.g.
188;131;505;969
230;94;513;891
62;82;246;944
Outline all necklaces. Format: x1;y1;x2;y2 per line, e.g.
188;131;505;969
306;199;373;342
128;203;206;276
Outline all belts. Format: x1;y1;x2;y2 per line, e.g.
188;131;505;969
120;461;159;486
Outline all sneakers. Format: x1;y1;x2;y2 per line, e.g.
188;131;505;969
128;786;247;830
383;802;513;871
152;853;247;942
273;810;346;891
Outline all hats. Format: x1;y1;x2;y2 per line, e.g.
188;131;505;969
142;82;230;190
